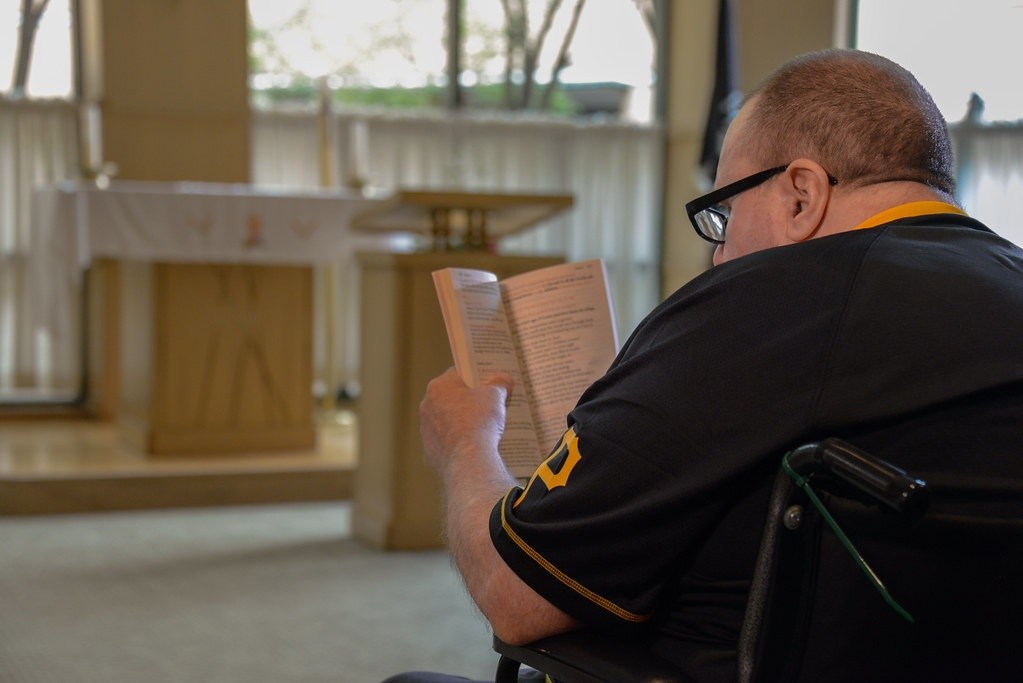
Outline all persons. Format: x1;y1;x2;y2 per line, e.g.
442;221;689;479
420;50;1023;682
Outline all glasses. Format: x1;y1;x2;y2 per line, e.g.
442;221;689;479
686;164;838;246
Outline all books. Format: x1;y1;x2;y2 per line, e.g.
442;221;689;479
432;257;622;478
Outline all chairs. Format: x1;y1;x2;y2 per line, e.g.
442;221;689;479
479;433;1023;683
